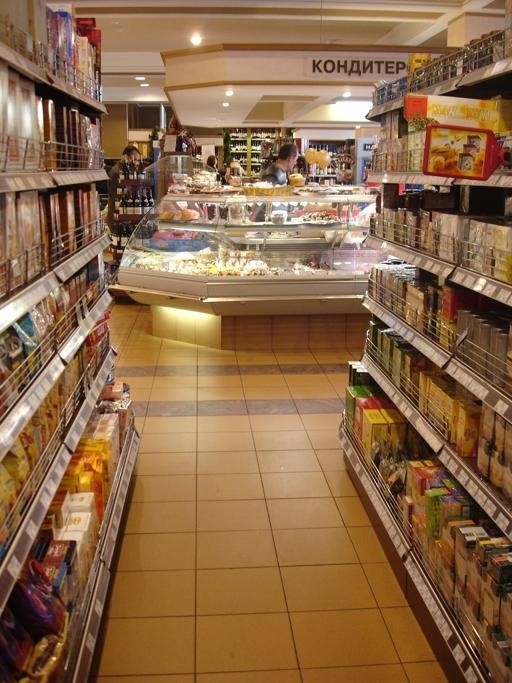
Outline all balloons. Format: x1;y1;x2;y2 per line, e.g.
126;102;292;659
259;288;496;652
313;150;333;170
305;147;318;166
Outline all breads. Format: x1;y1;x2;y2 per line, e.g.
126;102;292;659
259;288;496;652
161;208;200;221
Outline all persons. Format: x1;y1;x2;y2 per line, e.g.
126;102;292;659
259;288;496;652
251;142;299;222
204;155;226;180
104;144;142;236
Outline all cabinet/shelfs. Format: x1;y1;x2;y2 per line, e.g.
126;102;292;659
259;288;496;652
106;131;376;317
337;28;511;682
0;0;142;683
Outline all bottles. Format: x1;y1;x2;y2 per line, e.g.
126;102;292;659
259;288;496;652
316;161;346;175
110;159;154;249
230;128;272;177
